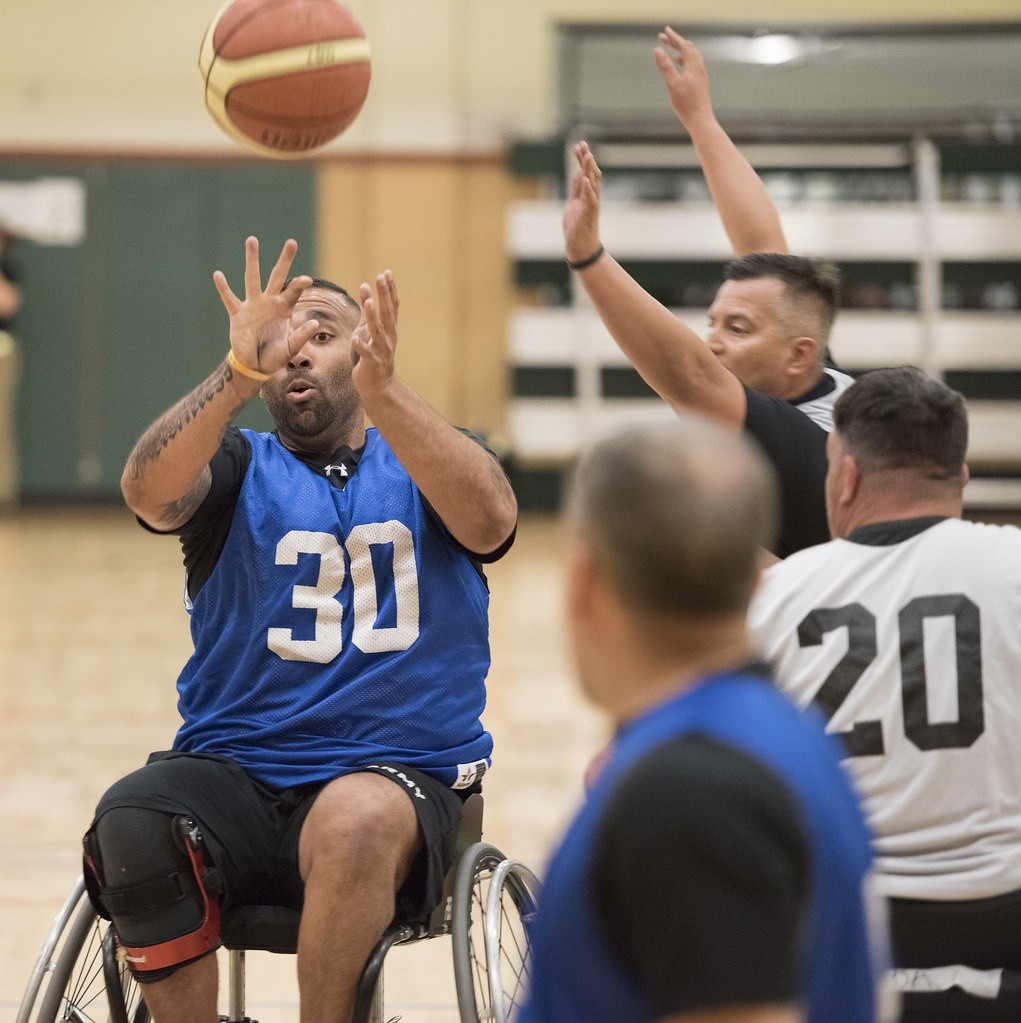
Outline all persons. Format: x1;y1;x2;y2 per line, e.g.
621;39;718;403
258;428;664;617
515;366;1021;1022
560;24;859;561
80;233;519;1023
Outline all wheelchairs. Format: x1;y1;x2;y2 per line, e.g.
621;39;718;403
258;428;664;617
15;793;540;1022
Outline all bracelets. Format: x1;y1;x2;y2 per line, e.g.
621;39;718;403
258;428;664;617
564;243;604;270
228;349;270;382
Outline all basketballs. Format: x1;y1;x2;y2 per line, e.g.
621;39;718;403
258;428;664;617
197;1;374;156
